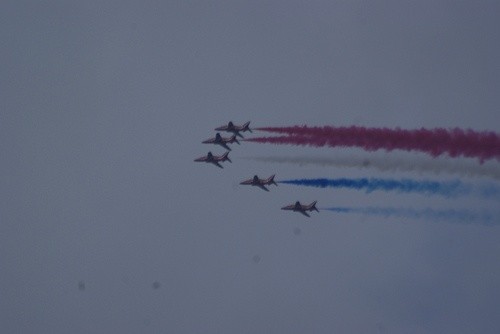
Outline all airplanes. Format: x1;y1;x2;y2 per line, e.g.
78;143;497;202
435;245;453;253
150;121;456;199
213;120;250;138
281;200;320;218
240;174;278;192
193;150;232;169
201;132;240;151
